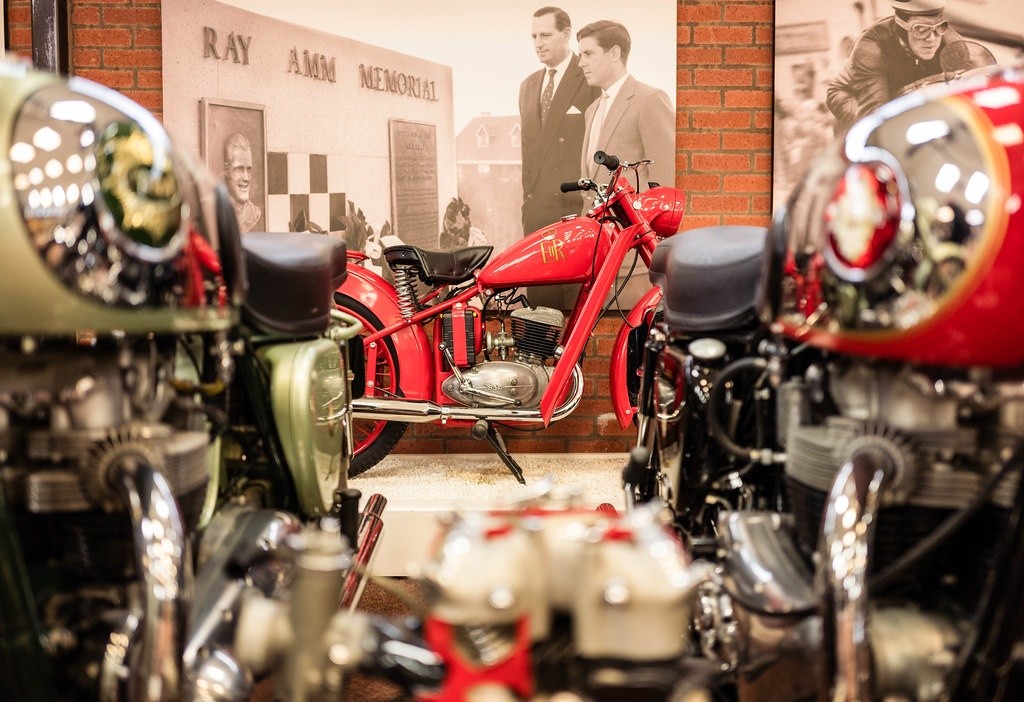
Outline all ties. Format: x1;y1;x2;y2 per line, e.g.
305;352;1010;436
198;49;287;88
586;93;610;178
538;68;557;125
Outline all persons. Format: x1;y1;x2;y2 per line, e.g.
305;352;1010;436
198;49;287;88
519;6;602;310
223;132;261;233
776;64;834;181
827;0;970;129
577;20;675;310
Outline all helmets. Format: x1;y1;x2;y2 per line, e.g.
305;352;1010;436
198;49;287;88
892;0;946;15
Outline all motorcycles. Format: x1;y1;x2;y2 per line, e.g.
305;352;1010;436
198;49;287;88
2;45;1024;701
322;149;686;478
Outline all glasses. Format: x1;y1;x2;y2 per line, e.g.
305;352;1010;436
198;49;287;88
894;13;949;38
